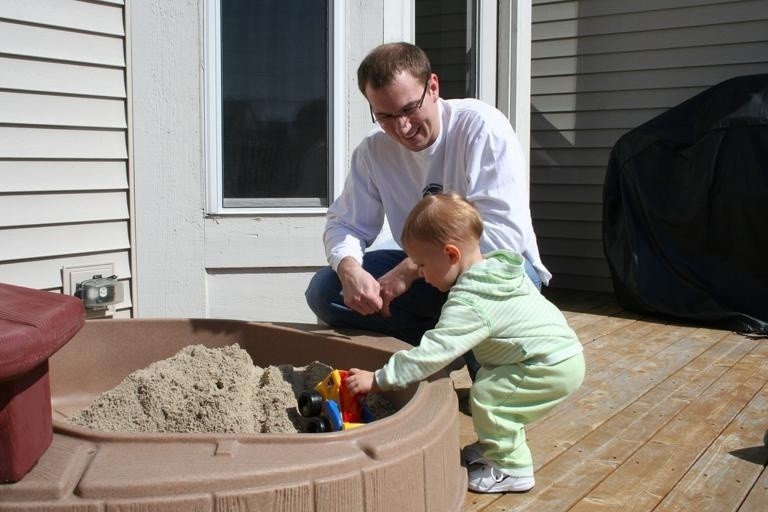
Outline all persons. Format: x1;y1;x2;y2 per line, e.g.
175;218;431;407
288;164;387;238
343;189;588;496
302;42;553;417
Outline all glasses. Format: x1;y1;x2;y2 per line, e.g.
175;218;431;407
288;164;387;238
367;76;434;127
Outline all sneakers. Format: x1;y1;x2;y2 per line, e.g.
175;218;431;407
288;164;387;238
462;438;488;463
466;463;537;495
